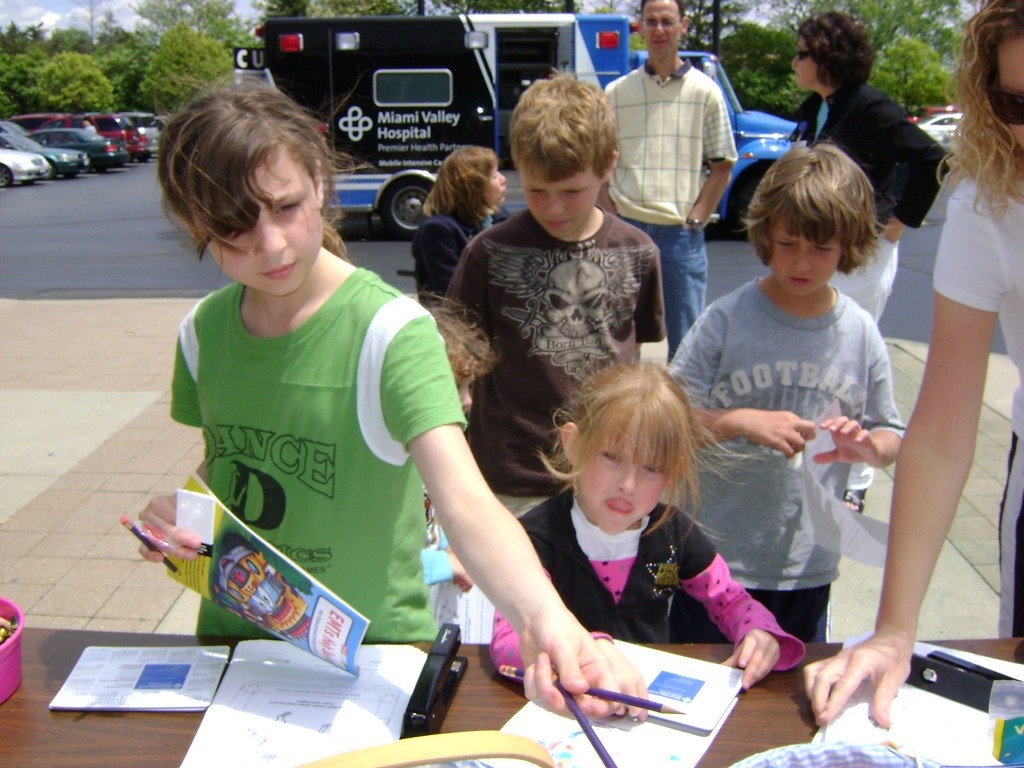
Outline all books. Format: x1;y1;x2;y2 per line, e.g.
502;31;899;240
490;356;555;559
166;473;371;677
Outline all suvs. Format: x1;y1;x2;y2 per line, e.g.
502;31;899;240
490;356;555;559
6;110;170;163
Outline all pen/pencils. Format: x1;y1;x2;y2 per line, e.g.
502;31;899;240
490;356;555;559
118;516;183;577
550;668;619;768
500;663;689;716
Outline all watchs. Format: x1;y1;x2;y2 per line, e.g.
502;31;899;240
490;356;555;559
686;218;704;232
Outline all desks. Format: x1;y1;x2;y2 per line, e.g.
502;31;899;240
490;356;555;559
0;626;1024;768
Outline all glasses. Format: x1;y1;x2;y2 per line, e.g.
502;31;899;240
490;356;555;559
794;51;812;61
984;65;1024;125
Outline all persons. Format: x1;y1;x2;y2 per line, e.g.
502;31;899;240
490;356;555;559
426;76;669;632
598;0;739;358
491;364;808;722
669;146;904;646
804;0;1024;727
792;11;947;514
411;146;509;307
139;86;623;722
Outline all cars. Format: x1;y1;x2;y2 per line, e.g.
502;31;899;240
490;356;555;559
0;146;53;188
905;106;969;150
0;121;91;178
25;128;131;174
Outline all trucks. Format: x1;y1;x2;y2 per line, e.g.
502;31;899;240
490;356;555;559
257;11;799;241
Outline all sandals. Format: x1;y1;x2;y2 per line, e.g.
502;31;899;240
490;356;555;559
843;489;866;514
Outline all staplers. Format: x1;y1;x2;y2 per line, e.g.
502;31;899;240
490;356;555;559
907;648;1023;715
402;622;470;739
